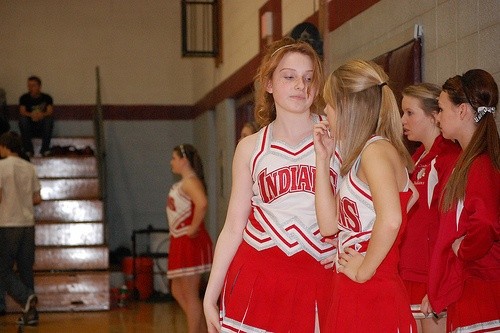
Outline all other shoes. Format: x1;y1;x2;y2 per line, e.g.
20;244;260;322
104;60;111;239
15;295;39;325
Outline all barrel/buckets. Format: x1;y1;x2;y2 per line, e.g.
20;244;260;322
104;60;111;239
124;257;154;297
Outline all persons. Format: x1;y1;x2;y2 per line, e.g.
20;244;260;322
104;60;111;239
314;58;420;333
164;143;213;333
422;69;500;333
0;88;41;325
17;75;54;157
204;37;344;333
401;83;466;333
